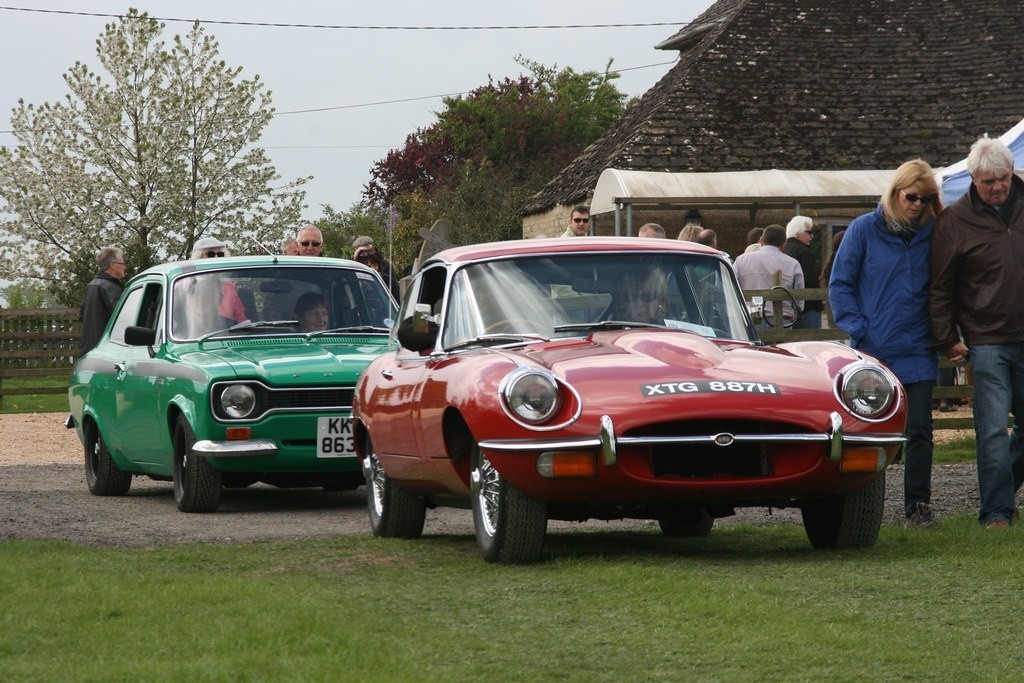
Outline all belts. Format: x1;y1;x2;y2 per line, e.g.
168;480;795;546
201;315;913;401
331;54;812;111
766;316;793;319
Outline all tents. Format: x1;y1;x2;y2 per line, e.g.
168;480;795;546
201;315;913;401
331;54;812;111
936;118;1024;206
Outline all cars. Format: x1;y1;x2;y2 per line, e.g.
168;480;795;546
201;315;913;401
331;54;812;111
346;235;908;564
58;255;409;508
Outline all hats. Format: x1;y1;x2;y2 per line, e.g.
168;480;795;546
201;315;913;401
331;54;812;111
193;238;226;252
354;245;384;264
351;236;373;248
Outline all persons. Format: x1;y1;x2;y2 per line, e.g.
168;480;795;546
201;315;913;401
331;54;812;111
454;275;526;336
638;212;825;329
931;138;1024;531
259;224;424;324
80;247;127;356
613;268;666;329
183;236;232;337
294;292;330;334
179;277;241;338
548;204;610;325
827;159;946;533
820;230;845;282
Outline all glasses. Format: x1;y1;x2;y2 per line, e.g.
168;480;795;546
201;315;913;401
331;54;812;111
113;262;127;267
805;230;812;235
900;190;931;204
298;241;321;247
571;218;589;223
202;251;224;258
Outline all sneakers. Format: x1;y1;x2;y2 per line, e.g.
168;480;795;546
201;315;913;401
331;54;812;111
905;502;935;531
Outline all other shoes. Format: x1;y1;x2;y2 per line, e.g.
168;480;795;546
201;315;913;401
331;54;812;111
986;521;1009;529
1011;508;1020;520
